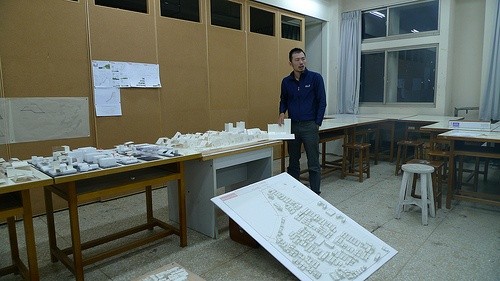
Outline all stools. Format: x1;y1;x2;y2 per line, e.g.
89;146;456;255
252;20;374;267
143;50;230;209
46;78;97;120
422;146;451;187
394;138;424;177
406;158;445;210
395;163;436;227
340;142;372;183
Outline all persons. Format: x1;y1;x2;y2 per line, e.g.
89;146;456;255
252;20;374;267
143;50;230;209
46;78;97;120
278;48;327;195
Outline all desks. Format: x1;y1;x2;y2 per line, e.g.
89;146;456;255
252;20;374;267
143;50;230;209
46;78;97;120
436;129;500;210
167;127;284;240
21;144;203;281
280;118;385;185
418;118;500;195
0;157;54;281
324;111;421;161
399;114;463;141
